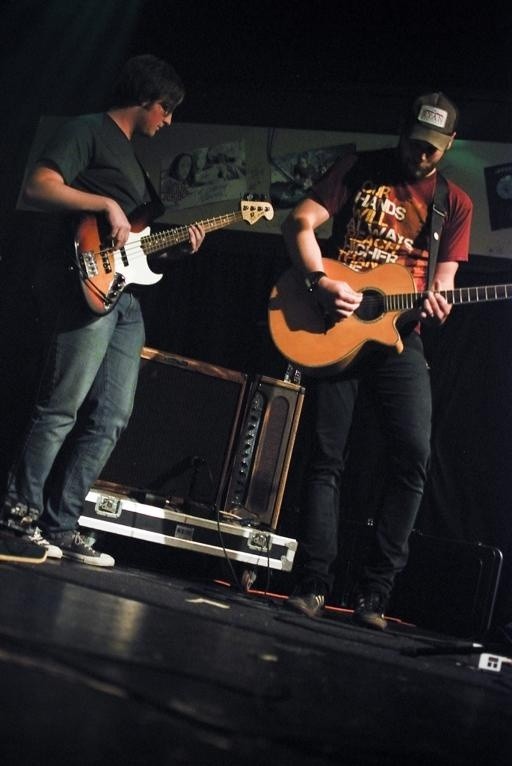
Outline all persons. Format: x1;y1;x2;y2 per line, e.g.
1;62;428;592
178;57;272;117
0;55;205;569
279;89;473;630
162;152;195;201
288;157;328;194
202;144;245;180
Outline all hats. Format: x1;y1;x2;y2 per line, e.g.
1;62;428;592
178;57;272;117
405;91;460;152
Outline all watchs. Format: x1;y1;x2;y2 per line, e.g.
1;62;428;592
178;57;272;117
301;271;324;295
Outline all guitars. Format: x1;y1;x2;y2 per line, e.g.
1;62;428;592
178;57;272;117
267;255;511;375
57;194;276;315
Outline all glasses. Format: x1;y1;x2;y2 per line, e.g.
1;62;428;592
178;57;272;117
163;102;173;116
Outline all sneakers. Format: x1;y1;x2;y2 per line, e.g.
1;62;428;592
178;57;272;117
283;595;324;618
28;527;63;561
44;529;115;567
354;593;388;630
